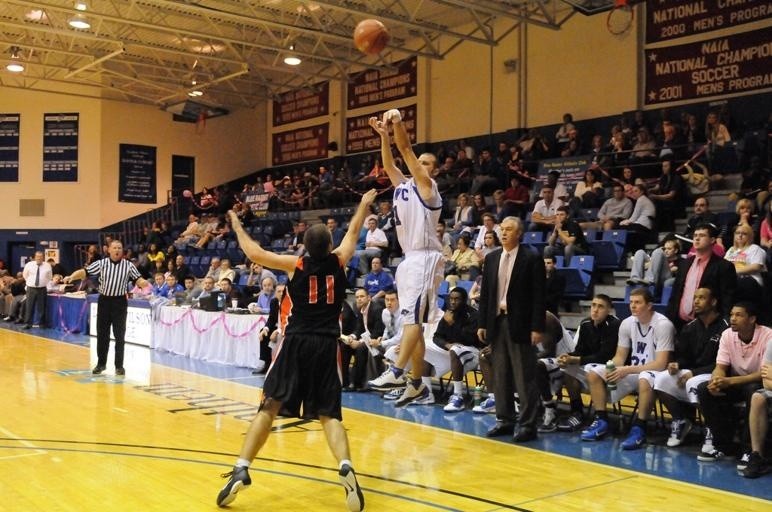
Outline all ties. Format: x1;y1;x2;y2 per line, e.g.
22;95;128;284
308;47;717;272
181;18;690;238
684;256;700;315
498;254;509;299
35;266;39;286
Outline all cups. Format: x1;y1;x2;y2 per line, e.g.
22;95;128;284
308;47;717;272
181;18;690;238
231;297;239;311
170;296;178;306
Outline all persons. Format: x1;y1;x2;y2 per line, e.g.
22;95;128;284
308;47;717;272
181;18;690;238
337;105;771;478
1;162;337;377
214;187;379;510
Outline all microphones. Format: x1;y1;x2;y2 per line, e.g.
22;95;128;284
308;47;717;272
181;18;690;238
264;293;268;296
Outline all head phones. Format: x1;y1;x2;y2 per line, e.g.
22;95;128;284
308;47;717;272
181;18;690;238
261;277;276;290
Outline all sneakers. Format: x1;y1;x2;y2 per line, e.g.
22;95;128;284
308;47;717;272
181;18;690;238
473;397;495;414
667;419;693;446
444;394;465;412
737;451;769;476
698;427;724;462
621;427;647;450
368;367;435;406
625;278;650;286
115;367;125;374
339;464;364;512
93;366;105;374
217;467;251;507
536;411;610;440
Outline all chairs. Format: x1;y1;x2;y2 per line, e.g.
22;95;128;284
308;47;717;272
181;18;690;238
90;131;772;448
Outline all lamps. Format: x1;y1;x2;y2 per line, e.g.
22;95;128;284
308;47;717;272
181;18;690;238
6;46;24;72
283;41;302;66
68;2;91;29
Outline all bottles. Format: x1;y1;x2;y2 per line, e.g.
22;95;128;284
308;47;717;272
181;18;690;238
474;384;482;405
604;359;616;392
361;331;379;356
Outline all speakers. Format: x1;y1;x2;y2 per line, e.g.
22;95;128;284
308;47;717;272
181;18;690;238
199;291;226;311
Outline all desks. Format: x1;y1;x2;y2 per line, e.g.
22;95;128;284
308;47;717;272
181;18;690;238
31;290;89;335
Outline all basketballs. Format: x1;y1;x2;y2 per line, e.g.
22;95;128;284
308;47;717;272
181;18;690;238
354;19;389;56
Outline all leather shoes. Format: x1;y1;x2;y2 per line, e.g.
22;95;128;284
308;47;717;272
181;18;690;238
513;434;536;443
252;368;266;374
488;426;509;436
